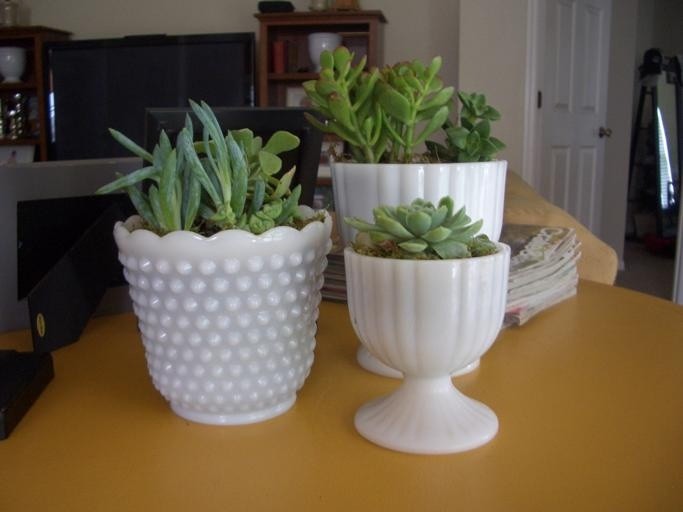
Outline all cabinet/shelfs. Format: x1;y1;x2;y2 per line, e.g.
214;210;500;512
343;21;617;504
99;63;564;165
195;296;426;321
0;23;73;161
254;9;388;186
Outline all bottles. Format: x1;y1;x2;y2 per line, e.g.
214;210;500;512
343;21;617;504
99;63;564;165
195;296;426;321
28;97;41;140
0;1;21;27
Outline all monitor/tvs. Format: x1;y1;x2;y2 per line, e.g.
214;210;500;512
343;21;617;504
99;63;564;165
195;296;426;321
142;106;325;209
43;33;254;160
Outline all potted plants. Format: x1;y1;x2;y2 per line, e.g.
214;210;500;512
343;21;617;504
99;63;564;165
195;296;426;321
94;97;333;427
337;194;513;457
299;44;508;381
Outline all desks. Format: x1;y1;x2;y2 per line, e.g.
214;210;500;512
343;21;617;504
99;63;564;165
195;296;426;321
0;278;683;511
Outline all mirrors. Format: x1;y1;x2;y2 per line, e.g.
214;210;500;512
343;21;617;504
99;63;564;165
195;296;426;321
653;72;682;239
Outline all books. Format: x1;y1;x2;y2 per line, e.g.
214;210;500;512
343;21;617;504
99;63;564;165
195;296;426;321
321;222;583;329
272;34;287;75
